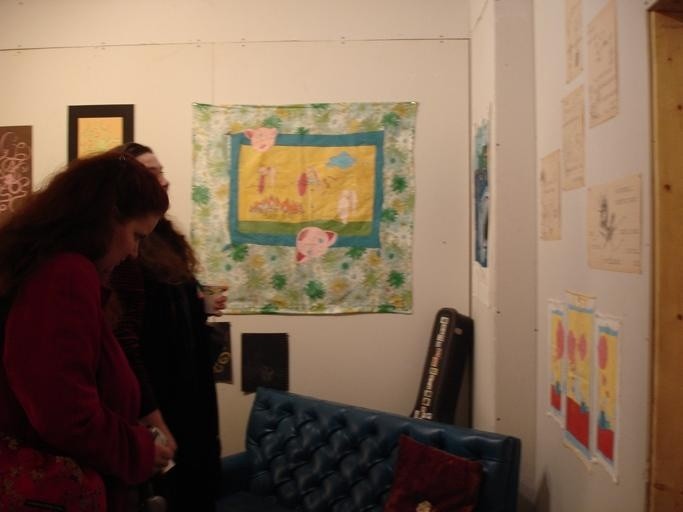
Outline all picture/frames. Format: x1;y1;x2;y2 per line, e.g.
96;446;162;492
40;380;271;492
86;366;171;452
67;104;135;165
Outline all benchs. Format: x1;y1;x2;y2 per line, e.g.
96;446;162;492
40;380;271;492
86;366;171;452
138;385;522;511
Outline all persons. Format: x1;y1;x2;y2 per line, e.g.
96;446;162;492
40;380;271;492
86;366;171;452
0;147;174;510
106;141;231;510
